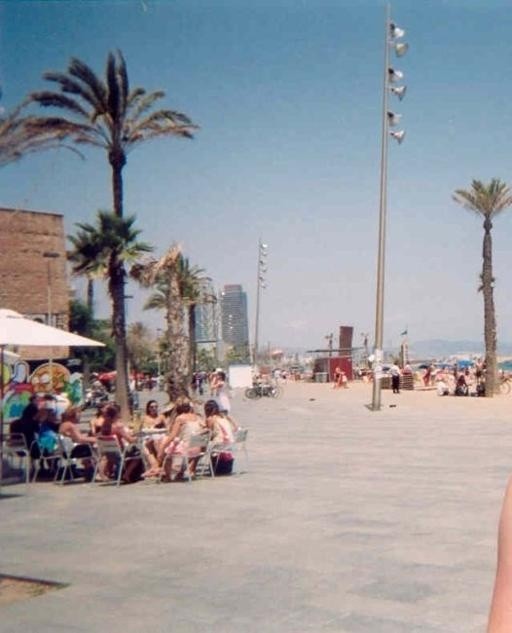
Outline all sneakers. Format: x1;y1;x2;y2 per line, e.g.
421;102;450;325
182;469;194;479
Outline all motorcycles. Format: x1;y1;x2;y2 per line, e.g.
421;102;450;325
242;379;281;398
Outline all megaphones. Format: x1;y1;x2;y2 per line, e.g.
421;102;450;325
386;22;409;145
258;242;267;289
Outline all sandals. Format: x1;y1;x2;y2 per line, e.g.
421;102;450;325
140;467;167;478
149;405;158;409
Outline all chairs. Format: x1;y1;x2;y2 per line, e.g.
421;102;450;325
0;425;249;488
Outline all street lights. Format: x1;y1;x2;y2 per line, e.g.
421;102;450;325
361;331;369;351
39;247;60;389
252;236;270;368
324;333;333;355
370;2;410;410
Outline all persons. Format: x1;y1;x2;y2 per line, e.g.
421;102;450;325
334;352;486;396
13;368;233;483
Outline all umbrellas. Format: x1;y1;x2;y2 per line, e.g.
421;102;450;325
1;307;107;348
